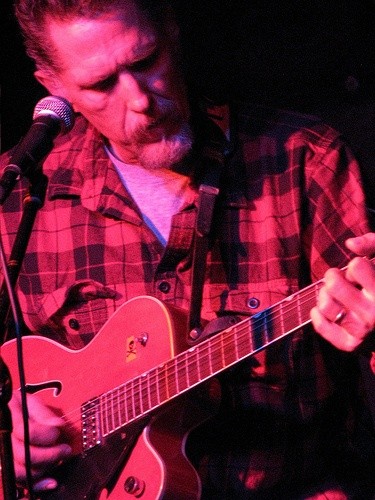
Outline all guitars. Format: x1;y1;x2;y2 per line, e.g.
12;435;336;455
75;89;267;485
0;254;374;500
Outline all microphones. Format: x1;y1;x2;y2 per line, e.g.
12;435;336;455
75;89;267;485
0;97;75;206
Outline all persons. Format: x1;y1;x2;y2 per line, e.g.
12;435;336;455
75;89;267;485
0;0;375;500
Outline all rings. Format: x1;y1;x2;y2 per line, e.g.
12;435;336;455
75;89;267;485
333;310;347;324
